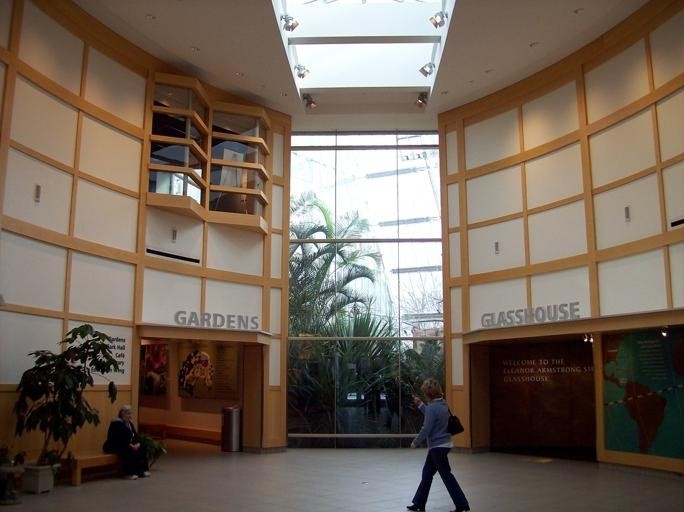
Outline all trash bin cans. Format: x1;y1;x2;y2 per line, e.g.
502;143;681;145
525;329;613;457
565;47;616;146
223;406;241;452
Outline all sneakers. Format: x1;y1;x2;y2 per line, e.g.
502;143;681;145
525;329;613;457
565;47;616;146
123;469;151;480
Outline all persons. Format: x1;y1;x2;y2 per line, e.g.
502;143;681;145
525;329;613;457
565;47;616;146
102;404;151;479
403;377;471;511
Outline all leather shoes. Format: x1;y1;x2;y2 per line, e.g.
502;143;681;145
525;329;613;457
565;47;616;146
406;504;425;512
450;504;470;512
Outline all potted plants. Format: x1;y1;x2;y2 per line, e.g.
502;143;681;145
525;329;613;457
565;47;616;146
13;324;124;495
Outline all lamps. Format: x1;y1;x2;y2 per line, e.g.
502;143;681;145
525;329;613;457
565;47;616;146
280;15;299;31
414;92;427;108
294;64;311;79
419;63;435;77
303;94;319;112
429;8;448;29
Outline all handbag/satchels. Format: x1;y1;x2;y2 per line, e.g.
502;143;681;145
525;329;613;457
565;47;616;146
448;415;465;436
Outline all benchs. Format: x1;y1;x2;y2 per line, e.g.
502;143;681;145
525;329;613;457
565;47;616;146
72;452;122;487
162;424;222;441
